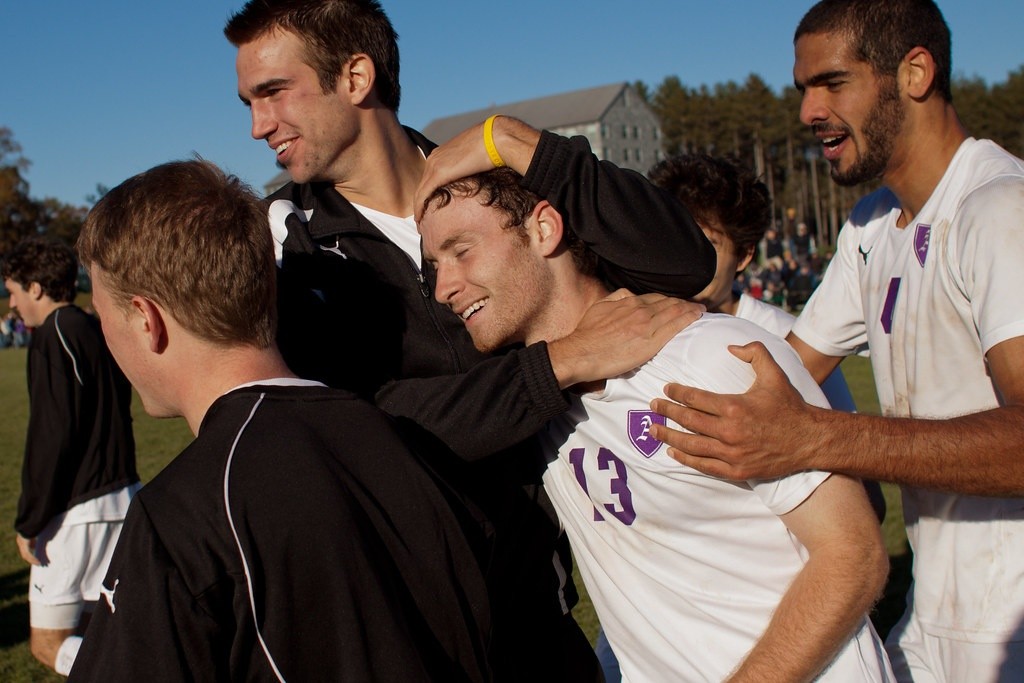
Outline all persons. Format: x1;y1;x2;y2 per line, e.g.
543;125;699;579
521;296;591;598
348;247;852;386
0;2;1023;683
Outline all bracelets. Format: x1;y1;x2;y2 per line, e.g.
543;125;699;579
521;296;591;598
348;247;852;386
483;113;505;168
